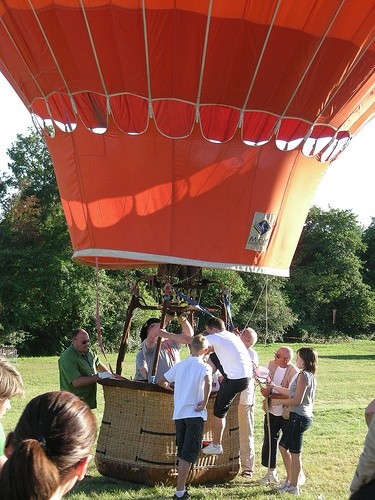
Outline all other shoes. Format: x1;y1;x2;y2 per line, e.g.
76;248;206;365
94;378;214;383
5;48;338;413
173;490;191;500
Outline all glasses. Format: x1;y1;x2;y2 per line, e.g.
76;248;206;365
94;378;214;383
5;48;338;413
274;353;280;359
76;339;91;345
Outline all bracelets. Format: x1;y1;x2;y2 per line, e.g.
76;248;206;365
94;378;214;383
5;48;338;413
277;399;281;405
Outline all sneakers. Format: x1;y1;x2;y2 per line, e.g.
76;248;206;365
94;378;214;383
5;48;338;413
242;470;253;478
298;472;306;485
275;480;299;495
202;442;223;455
257;474;279;482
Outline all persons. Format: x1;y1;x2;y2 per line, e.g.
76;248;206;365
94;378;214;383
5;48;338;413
260;346;307;488
58;328;125;411
266;347;319;495
156;317;253;455
240;327;259;479
157;335;213;500
132;316;209;385
348;399;375;500
0;391;97;500
0;357;26;471
